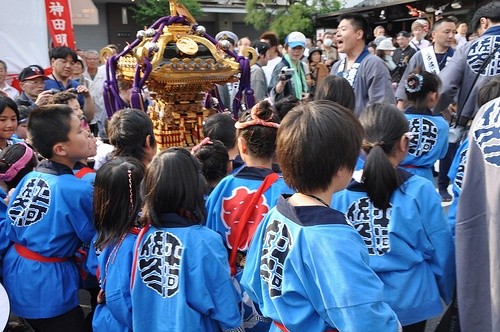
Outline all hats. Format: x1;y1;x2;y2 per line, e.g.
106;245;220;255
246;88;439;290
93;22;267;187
19;65;50;82
285;31;306;49
376;40;396;51
215;31;238;46
397;31;410;37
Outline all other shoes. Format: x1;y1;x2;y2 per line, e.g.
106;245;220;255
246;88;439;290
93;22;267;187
440;190;452;201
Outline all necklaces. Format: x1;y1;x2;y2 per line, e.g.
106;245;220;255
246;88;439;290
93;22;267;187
437;52;448;65
302;191;331;207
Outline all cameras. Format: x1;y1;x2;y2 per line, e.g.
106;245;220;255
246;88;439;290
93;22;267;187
279;68;296;80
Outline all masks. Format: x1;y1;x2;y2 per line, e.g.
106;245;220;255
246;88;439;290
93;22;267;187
323;39;333;47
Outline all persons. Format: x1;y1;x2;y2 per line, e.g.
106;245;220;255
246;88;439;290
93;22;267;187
0;2;500;332
